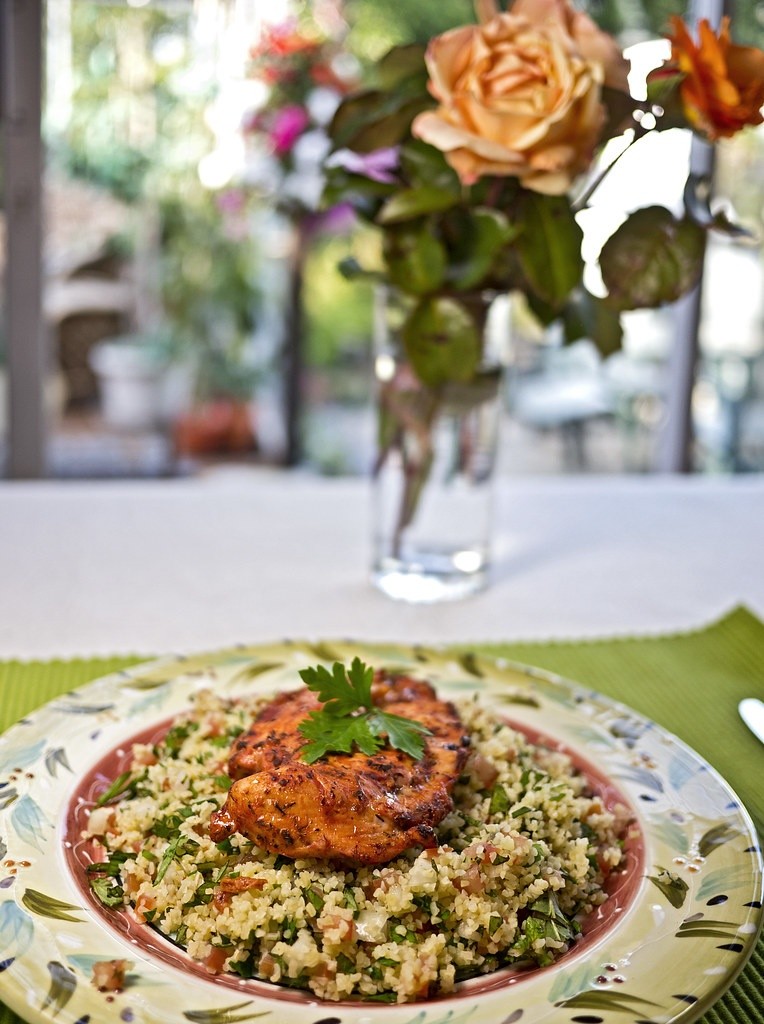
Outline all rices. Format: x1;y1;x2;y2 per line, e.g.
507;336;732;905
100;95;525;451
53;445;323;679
80;658;630;1005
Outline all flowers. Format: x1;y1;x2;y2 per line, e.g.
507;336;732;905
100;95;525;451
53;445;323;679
317;1;764;563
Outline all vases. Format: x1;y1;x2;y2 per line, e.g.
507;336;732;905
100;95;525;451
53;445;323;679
364;284;515;607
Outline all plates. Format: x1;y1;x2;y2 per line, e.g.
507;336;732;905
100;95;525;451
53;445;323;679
1;639;762;1024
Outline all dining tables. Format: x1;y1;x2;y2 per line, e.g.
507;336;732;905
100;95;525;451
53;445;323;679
0;481;763;1024
